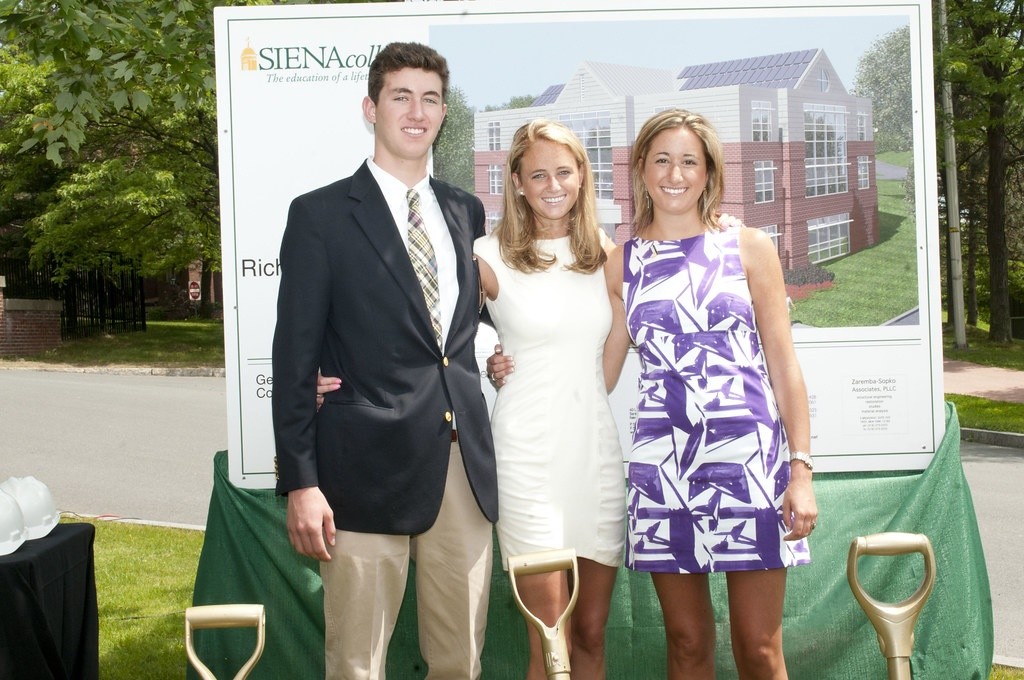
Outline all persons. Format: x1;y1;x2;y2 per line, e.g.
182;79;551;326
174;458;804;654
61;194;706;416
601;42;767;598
786;291;797;317
317;120;627;680
271;40;488;680
485;109;817;680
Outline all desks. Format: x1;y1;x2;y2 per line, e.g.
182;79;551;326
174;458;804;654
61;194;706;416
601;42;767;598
0;523;101;680
185;400;997;680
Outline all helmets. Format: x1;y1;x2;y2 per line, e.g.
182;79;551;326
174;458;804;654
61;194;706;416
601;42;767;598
2;476;60;540
0;489;27;556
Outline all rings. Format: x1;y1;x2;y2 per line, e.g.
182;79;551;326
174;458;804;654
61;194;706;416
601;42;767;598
492;372;497;381
810;521;816;530
317;394;323;398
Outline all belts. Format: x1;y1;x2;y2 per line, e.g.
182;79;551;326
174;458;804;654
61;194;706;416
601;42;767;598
450;429;457;442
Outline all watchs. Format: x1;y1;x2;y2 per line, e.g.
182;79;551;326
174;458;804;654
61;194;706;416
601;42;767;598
790;451;814;470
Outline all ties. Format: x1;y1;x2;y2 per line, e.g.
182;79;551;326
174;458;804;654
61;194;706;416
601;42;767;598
407;189;442;354
